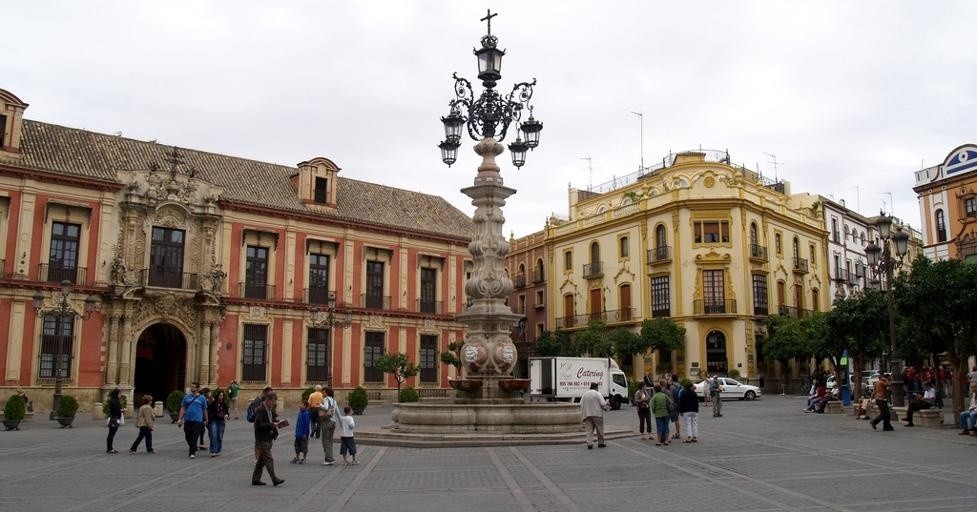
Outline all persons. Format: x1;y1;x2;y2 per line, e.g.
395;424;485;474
633;369;723;444
16;386;30;405
579;383;609;449
861;363;977;437
105;383;361;486
802;369;839;413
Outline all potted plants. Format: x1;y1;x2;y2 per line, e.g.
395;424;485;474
346;387;368;415
167;390;184;423
1;394;25;431
57;395;80;428
302;387;317;409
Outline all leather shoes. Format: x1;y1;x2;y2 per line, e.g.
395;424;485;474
273;478;285;485
252;480;266;485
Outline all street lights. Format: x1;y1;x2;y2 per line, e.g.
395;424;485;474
438;5;545;404
863;205;909;406
308;293;353;390
29;280;101;421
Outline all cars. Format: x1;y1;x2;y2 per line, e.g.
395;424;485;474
688;376;764;402
826;368;893;393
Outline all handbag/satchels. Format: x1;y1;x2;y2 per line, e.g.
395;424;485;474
319;398;333;419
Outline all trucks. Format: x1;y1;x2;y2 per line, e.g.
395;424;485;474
526;356;629;411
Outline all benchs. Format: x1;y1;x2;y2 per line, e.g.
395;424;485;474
892;407;943;426
815;401;843;414
864;405;893;419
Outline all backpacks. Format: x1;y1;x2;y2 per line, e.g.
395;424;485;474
247;403;255;422
